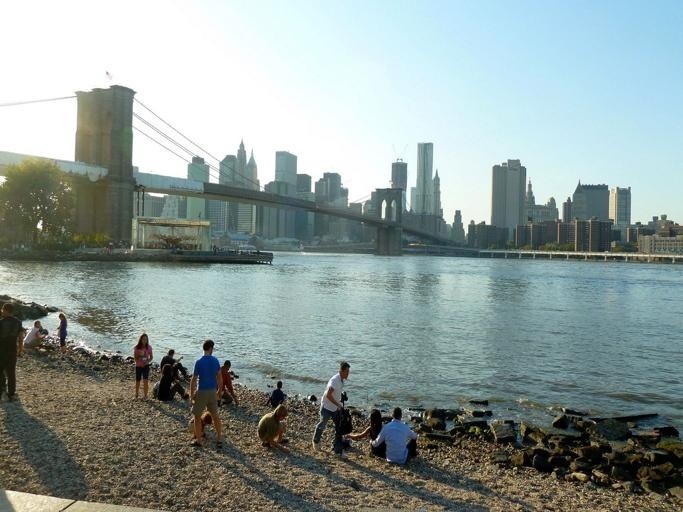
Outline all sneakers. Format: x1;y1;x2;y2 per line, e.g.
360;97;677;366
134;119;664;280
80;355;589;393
311;439;319;451
334;450;348;459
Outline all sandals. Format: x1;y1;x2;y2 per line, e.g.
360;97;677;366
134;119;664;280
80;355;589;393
216;440;222;448
186;439;203;447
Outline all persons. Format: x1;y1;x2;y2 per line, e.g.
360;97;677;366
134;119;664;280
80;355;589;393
221;360;239;405
261;380;285;408
133;333;153;401
369;407;418;466
157;363;189;402
188;340;224;450
160;350;192;380
57;314;68;354
341;408;386;460
257;404;291;454
23;320;47;349
312;361;351;452
0;303;24;400
